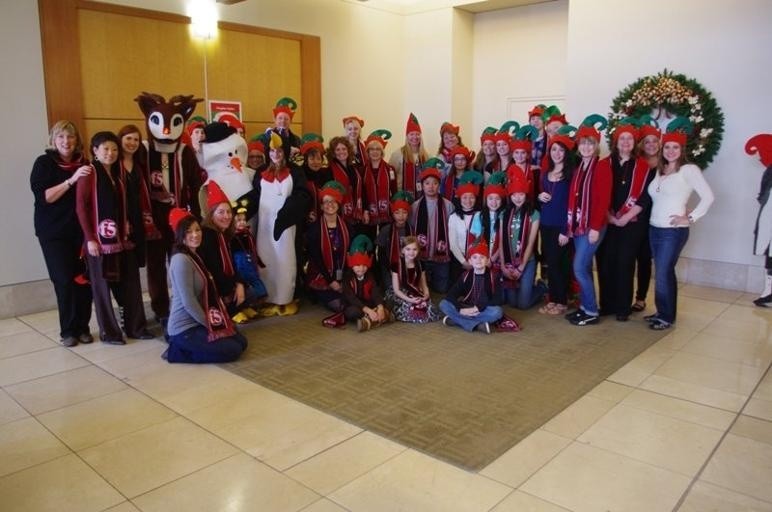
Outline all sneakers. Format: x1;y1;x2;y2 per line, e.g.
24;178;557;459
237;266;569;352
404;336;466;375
632;301;647;312
643;311;659;321
357;317;371;332
109;338;126;345
548;304;569;315
137;329;155;340
752;294;772;306
649;319;670;330
442;315;452;326
536;278;548;294
565;308;585;321
481;321;491;334
539;302;557;314
571;314;600;326
78;332;94;343
63;335;79;347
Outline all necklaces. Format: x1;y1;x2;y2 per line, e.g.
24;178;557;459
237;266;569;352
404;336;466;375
239;238;252;263
156;144;170;170
194;253;214;281
618;155;627;185
655;164;676;193
328;222;340;251
273;169;283;198
411;152;419;166
509;213;519;239
547;172;561;196
575;161;590;197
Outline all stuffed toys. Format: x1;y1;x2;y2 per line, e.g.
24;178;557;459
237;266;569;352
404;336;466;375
133;90;205;324
199;122;257;238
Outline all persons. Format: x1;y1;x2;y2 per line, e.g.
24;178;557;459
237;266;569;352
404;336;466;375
498;164;547;310
643;116;714;330
505;125;539;206
248;142;266;168
232;132;315;317
745;134;772;304
327;137;364;238
528;105;547;165
439;230;503;334
435;123;467;164
408;158;456;294
75;131;156;346
448;171;484;268
195;180;246;308
110;124;165;332
225;200;267;324
213;111;245;137
363;130;398;274
341;234;390;332
293;133;332;217
473;127;496;173
30;120;94;347
564;114;611;326
343;117;367;172
375;189;414;269
161;208;248;364
537;125;577;315
465;171;507;305
384;235;444;323
265;96;302;155
542;105;568;138
387;113;430;202
628;114;662;311
440;147;475;201
186;116;209;155
306;180;355;312
484;122;519;186
598;116;650;322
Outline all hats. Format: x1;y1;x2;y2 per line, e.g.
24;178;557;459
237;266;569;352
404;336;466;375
612;117;641;140
420;157;445;180
547;125;577;150
575;113;608;143
439;121;460;135
363;128;392;151
346;233;374;269
511;125;540;151
528;103;548;116
405;112;422;134
246;132;269;153
205;179;229;208
662;116;694;145
272;96;297;120
744;133;772;168
318;180;345;204
390;190;413;214
167;207;194;234
541;105;567;126
480;127;498;144
504;163;530;194
452;146;476;162
299;132;326;156
187;115;207;136
483;171;508;198
455;170;484;196
494;120;520;145
638;114;662;138
467;231;489;258
230;198;250;214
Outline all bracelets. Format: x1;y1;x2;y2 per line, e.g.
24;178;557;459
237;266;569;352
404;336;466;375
516;267;523;274
490;256;494;265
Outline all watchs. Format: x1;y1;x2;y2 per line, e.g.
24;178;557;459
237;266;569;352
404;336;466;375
688;215;695;224
66;180;72;187
